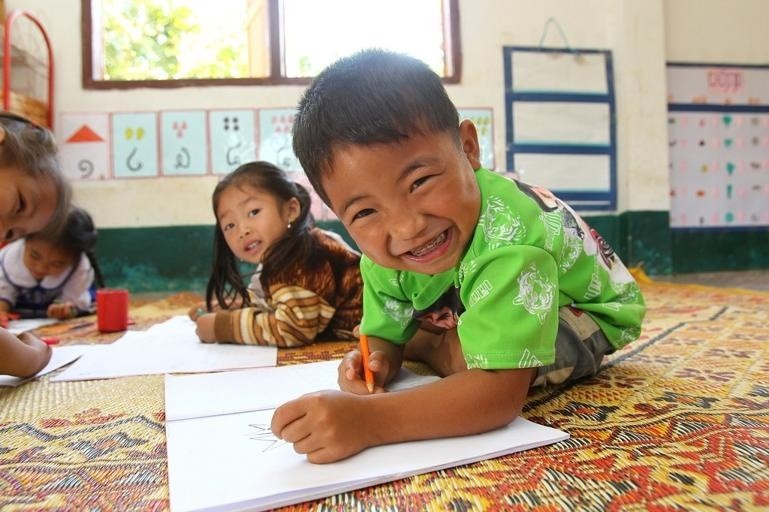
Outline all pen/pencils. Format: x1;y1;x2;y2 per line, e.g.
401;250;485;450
360;334;374;393
43;338;59;344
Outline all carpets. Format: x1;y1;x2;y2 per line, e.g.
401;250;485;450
0;269;769;512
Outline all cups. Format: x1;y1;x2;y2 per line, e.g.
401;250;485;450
96;288;130;334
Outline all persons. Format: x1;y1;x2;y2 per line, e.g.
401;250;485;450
188;161;363;350
0;204;105;318
0;111;74;379
269;47;648;464
